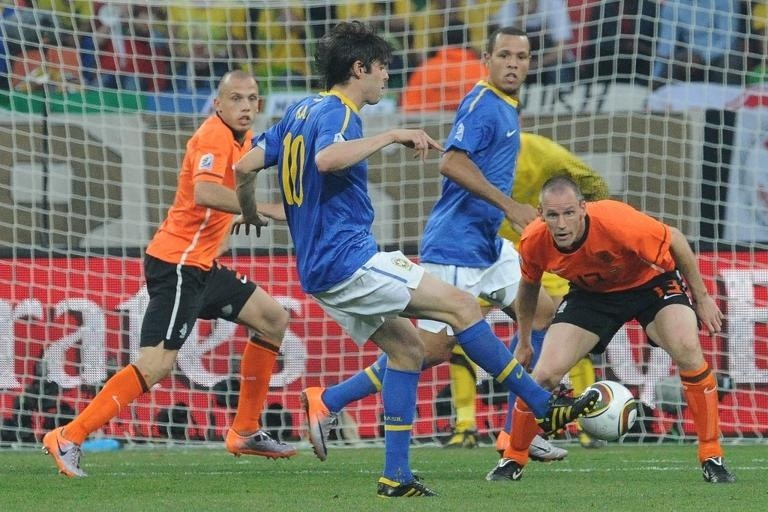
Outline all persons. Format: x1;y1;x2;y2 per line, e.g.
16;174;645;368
0;1;768;114
485;172;736;486
446;128;607;454
302;27;572;466
228;22;599;501
41;68;298;483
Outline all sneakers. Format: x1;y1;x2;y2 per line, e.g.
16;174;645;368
702;456;736;483
446;429;568;482
301;387;335;461
226;428;295;460
535;390;598;437
377;477;438;498
42;426;88;477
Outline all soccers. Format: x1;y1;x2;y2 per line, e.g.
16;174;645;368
577;381;637;442
